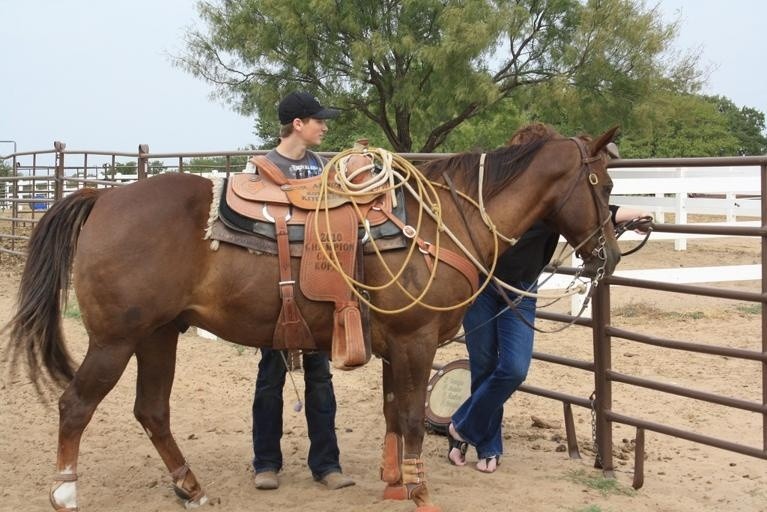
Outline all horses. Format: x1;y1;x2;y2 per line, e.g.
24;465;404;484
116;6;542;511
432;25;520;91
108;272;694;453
3;124;622;510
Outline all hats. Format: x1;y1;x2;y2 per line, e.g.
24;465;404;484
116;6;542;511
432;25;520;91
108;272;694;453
279;92;340;125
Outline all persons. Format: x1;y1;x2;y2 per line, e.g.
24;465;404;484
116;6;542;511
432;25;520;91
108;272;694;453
249;88;356;488
443;122;657;472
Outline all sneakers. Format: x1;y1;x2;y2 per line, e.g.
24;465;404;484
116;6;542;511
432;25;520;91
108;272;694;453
313;471;355;490
254;467;279;490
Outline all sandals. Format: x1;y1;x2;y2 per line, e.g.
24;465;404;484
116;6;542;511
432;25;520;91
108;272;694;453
446;421;469;466
475;454;498;473
